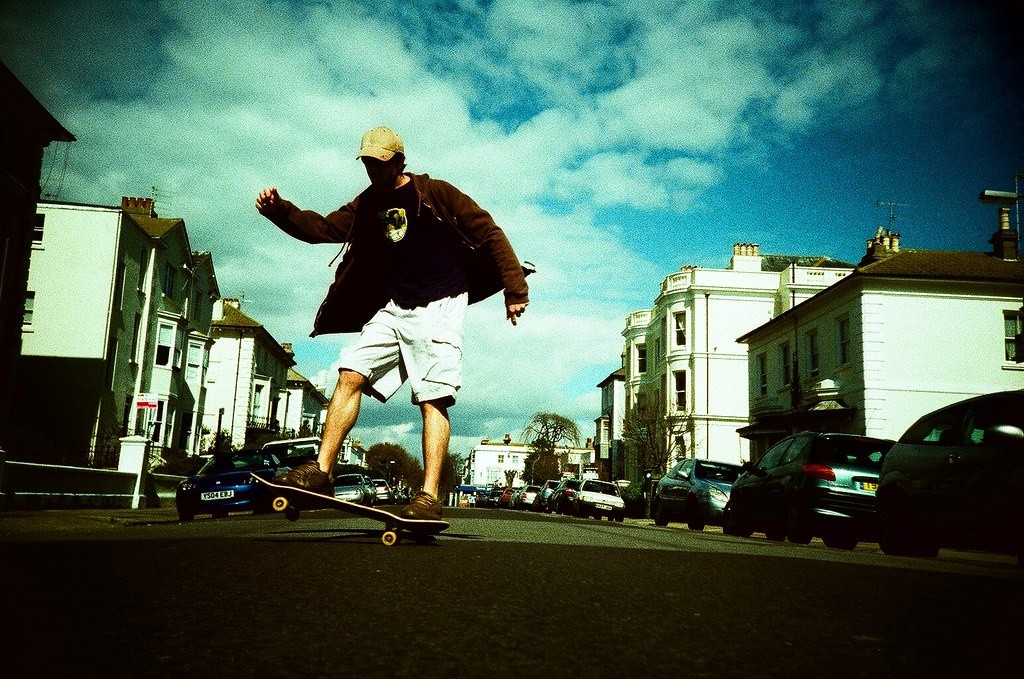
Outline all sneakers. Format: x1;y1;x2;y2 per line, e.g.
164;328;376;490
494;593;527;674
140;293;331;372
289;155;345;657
400;491;442;520
273;459;335;496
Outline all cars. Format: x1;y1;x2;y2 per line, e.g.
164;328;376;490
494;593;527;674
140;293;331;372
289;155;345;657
574;479;626;522
875;388;1024;557
498;488;517;509
363;477;378;505
650;458;743;532
518;485;541;510
535;480;562;513
176;450;291;521
333;473;369;506
549;478;582;514
509;489;523;509
371;479;393;504
477;491;503;508
722;429;898;550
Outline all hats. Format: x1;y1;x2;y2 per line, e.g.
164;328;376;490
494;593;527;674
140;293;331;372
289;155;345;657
355;126;404;161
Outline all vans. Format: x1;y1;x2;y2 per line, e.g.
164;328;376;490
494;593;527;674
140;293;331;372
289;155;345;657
261;437;323;469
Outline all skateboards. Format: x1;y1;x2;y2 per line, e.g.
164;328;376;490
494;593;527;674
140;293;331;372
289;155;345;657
249;470;450;547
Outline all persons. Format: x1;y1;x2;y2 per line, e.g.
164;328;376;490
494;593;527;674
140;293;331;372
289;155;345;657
257;128;530;520
